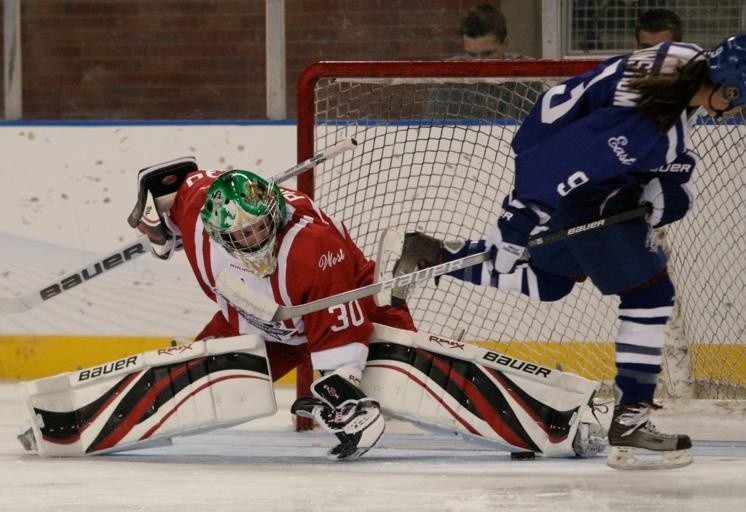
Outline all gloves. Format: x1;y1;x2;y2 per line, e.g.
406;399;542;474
485;189;553;278
638;149;700;229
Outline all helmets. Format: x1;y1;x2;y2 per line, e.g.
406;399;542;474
201;170;285;278
707;34;746;106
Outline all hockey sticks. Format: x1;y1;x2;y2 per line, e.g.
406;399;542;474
213;204;654;321
0;139;359;313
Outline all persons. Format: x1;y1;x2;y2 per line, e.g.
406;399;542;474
632;8;685;51
423;6;544;119
16;155;611;458
374;31;746;450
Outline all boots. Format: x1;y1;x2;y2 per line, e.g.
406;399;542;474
391;232;466;308
608;383;691;451
